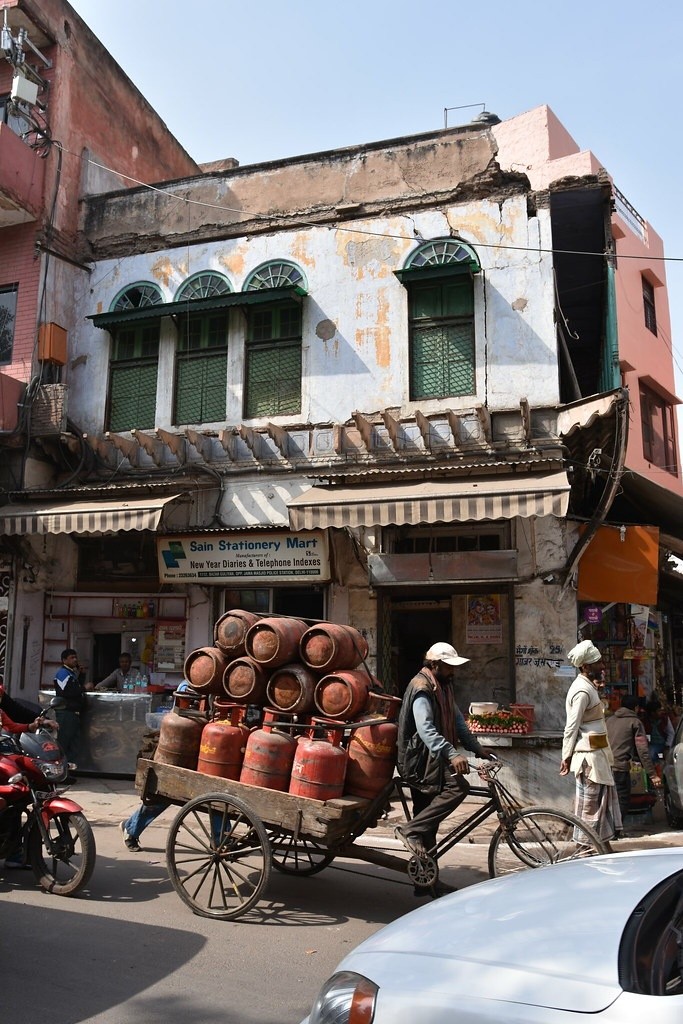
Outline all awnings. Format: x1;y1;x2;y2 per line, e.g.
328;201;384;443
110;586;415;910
0;492;189;535
287;470;572;531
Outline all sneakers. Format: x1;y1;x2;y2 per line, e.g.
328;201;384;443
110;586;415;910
210;842;249;857
119;820;142;852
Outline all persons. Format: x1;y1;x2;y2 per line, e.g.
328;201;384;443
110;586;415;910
53;649;93;784
120;647;232;852
606;696;663;840
559;640;623;857
95;653;141;688
640;700;675;762
395;643;499;898
0;688;59;869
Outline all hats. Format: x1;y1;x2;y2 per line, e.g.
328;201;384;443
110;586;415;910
567;639;601;668
425;642;471;666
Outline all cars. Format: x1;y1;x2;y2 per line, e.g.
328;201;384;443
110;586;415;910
294;844;683;1024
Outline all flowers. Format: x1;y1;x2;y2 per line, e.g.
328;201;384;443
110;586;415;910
467;710;527;730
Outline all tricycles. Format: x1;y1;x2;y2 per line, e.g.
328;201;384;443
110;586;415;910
131;747;614;924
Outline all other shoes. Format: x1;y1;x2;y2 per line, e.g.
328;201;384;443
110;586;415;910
5;854;32;870
61;777;77;785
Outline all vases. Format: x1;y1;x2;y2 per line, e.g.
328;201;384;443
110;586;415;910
468;717;528;735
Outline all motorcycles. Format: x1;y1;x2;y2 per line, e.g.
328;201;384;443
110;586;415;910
0;694;96;895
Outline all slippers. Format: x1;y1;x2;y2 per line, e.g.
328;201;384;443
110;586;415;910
414;881;458;897
395;827;428;863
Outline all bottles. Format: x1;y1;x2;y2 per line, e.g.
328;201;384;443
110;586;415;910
114;600;154;617
128;678;134;693
135;673;142;694
141;674;148;695
123;678;128;694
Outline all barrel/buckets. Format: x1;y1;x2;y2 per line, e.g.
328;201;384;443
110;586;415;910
509;703;538;731
469;702;499;715
